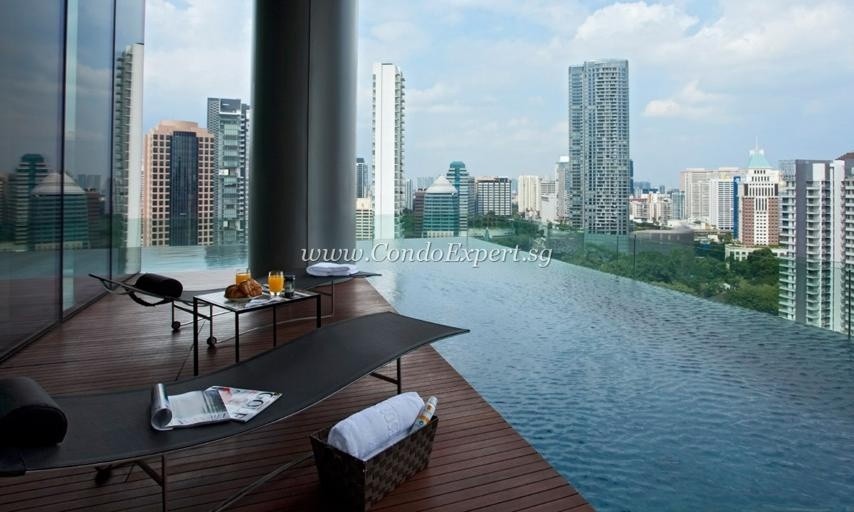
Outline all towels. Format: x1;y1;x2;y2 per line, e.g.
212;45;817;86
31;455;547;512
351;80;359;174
326;391;425;461
305;261;362;277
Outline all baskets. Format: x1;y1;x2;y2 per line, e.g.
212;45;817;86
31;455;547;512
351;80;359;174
309;401;439;511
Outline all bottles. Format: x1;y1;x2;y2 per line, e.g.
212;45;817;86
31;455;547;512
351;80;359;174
409;396;438;434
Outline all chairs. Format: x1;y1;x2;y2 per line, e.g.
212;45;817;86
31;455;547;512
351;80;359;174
87;253;381;348
0;309;473;512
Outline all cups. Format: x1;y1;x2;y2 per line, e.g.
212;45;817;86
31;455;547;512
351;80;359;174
267;270;283;296
283;275;294;297
235;268;251;284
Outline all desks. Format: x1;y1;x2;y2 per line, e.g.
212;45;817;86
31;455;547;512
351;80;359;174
191;282;323;377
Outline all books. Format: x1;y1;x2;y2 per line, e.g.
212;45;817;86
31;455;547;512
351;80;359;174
204;384;282;424
148;382;231;431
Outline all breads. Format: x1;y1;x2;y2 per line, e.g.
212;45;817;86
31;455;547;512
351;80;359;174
225;285;247;298
239;278;262;297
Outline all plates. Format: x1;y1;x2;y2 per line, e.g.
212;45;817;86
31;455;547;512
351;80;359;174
225;293;263;302
